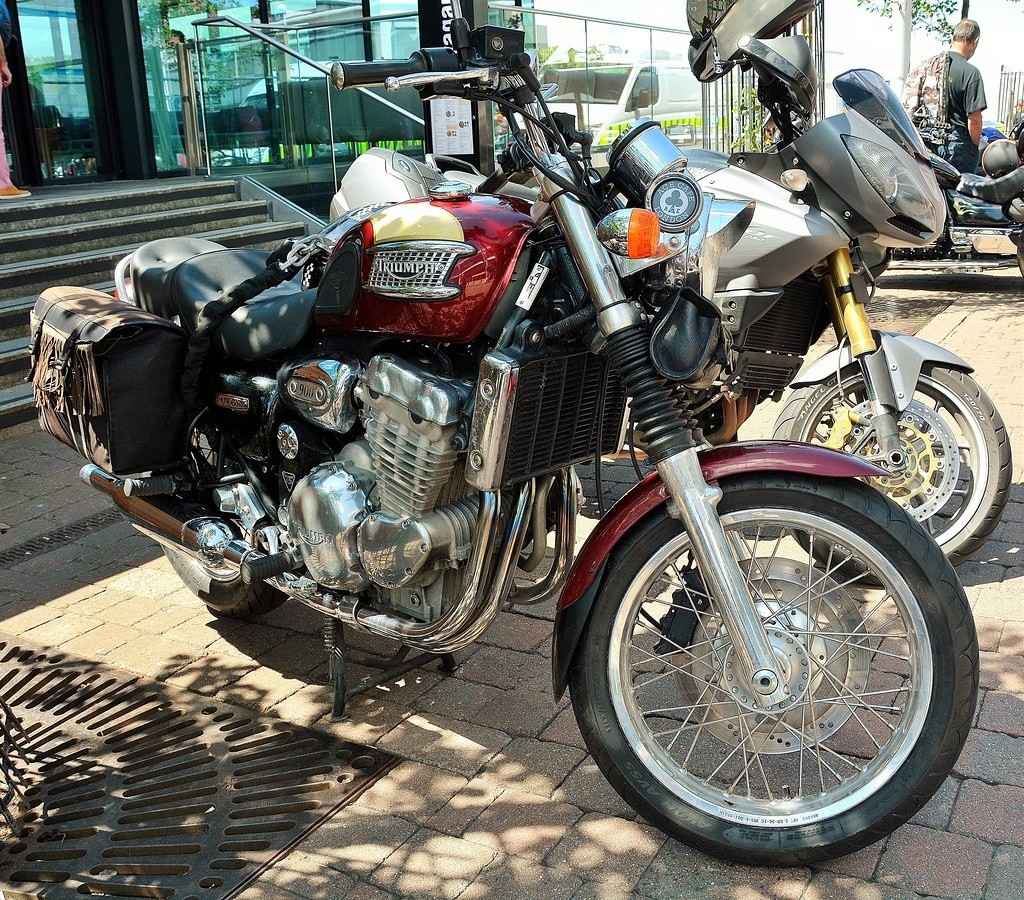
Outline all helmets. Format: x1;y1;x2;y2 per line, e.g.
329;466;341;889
686;1;817;83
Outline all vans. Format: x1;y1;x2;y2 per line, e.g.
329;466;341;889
512;60;747;153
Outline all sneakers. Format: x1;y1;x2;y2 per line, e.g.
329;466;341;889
0;184;32;200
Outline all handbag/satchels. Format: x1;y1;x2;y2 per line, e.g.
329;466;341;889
24;284;191;478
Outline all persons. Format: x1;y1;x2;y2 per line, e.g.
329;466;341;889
900;18;987;173
0;0;31;199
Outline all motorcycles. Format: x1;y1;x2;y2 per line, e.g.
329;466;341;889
24;0;1024;870
